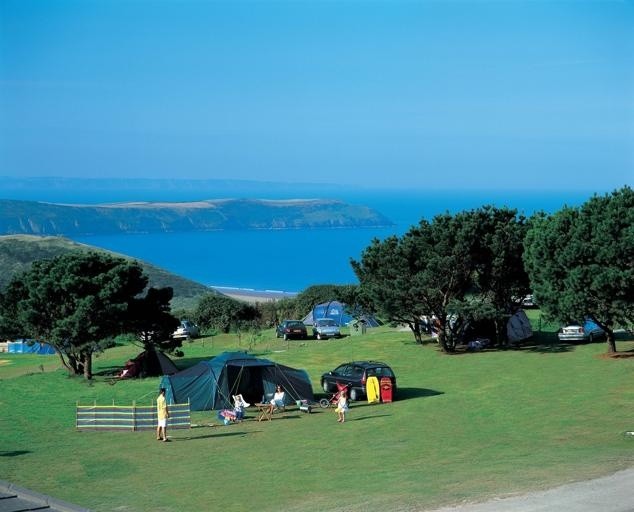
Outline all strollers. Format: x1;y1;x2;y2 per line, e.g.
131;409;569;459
318;381;353;409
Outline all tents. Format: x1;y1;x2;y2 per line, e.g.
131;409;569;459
159;351;316;411
301;300;384;328
117;350;180;378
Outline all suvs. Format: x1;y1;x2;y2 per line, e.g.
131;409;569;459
275;320;307;341
320;360;397;401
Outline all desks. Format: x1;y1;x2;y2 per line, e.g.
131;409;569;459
253;402;274;422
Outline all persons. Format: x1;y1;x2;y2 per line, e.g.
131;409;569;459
270;385;286;406
156;388;172;442
232;396;244;420
335;391;348;422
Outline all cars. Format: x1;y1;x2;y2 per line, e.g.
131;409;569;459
137;328;172;345
172;321;202;341
421;314;459;341
311;318;341;340
513;295;538;307
557;319;607;343
